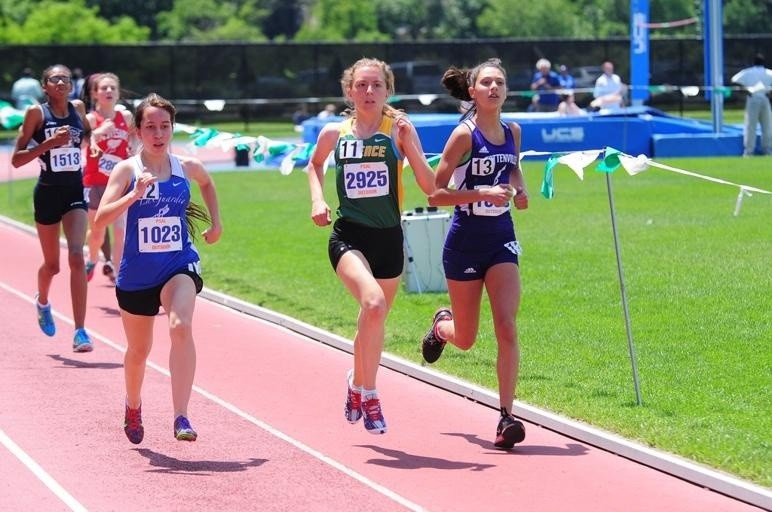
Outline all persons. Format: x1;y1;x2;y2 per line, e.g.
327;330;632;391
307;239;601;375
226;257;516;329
11;67;42;108
9;62;103;353
592;61;622;111
80;70;140;282
79;74;127;287
93;91;223;447
530;59;561;113
729;52;772;158
306;58;438;434
556;90;583;114
559;64;576;90
422;58;528;450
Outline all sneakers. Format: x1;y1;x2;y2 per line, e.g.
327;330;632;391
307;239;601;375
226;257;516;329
493;414;526;449
344;369;364;425
173;415;197;442
361;398;388;435
123;391;144;445
85;257;97;283
72;327;94;353
421;307;453;365
103;260;117;284
33;292;56;336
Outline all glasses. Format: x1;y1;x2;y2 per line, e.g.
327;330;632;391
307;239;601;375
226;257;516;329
46;75;73;85
